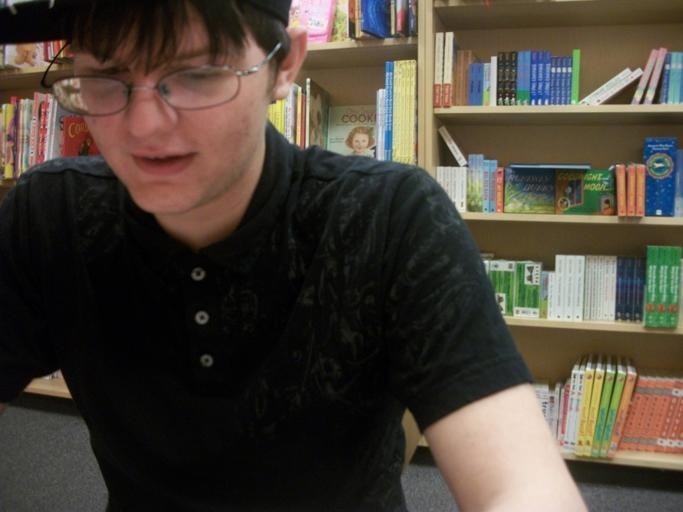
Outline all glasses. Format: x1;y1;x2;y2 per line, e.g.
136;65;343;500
41;42;283;117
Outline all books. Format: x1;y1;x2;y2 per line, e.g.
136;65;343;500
435;31;683;218
1;39;102;181
481;246;683;327
534;354;683;461
269;0;419;167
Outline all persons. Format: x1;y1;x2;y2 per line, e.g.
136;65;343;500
0;2;590;512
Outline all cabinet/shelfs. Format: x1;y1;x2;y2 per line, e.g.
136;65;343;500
426;1;683;472
